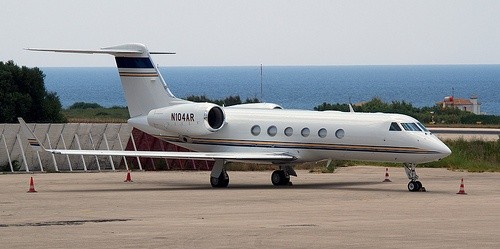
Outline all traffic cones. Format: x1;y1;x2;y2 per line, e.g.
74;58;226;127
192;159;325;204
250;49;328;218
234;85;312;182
27;177;37;193
382;168;392;183
456;178;468;195
124;173;134;183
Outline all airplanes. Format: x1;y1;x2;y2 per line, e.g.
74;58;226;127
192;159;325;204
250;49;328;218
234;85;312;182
18;43;451;191
